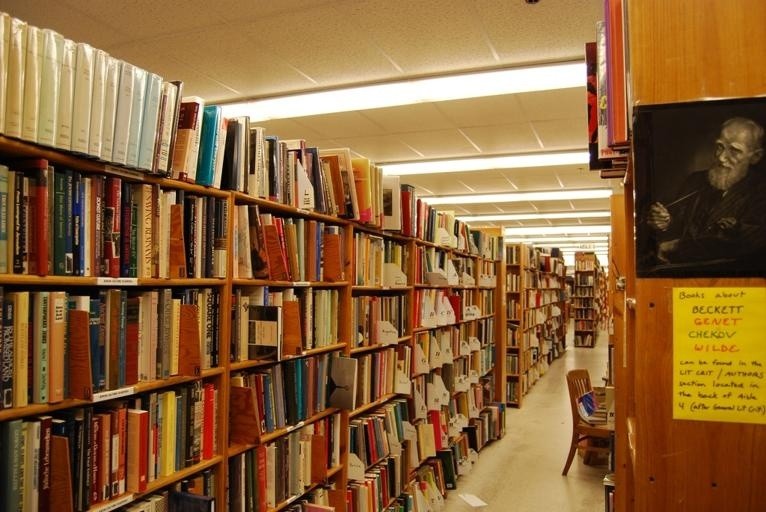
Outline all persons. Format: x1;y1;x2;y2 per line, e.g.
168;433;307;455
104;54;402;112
646;113;766;275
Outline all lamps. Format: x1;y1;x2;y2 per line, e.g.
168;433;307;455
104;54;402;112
370;148;606;266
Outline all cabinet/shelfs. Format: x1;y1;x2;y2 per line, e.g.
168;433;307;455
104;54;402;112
574;250;605;349
609;0;766;510
504;243;566;412
0;135;504;512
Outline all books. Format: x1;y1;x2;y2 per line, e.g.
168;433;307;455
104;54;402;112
225;116;412;512
2;12;226;512
401;184;508;511
570;251;610;346
585;0;629;179
505;243;571;406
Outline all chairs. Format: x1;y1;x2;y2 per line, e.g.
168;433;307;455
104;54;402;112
564;368;615;475
